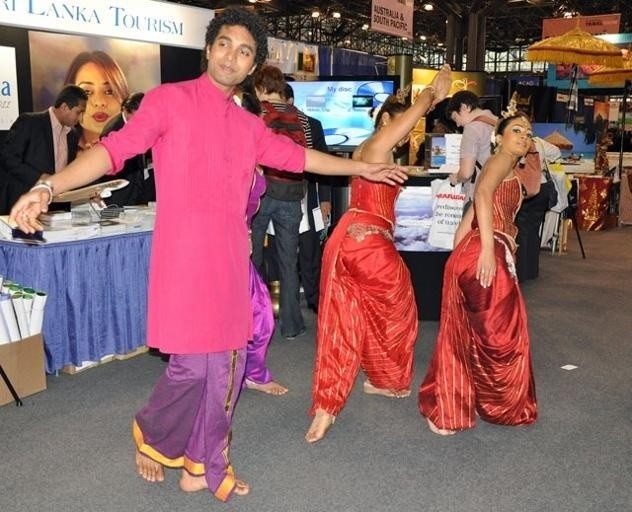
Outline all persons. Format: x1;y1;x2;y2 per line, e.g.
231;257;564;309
100;91;156;208
65;50;130;157
233;66;335;339
1;86;90;214
254;66;312;340
232;90;289;396
411;91;562;287
304;65;453;443
417;112;538;437
8;8;411;503
266;83;332;313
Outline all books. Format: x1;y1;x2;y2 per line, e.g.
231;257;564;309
0;200;156;245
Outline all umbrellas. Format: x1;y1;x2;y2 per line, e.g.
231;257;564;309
526;19;622;121
589;48;632;180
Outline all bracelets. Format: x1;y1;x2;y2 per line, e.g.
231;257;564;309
28;182;54;206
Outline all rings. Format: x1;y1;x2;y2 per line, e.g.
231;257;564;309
327;214;330;219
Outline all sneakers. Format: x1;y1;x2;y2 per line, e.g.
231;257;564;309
286;325;308;341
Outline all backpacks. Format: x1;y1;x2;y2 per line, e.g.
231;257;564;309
472;114;542;200
259;100;309;185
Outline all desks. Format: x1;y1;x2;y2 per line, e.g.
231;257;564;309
0;203;156;376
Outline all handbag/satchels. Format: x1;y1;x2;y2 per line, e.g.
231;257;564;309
428;177;466;251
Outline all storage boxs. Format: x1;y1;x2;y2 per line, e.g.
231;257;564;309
0;330;48;407
